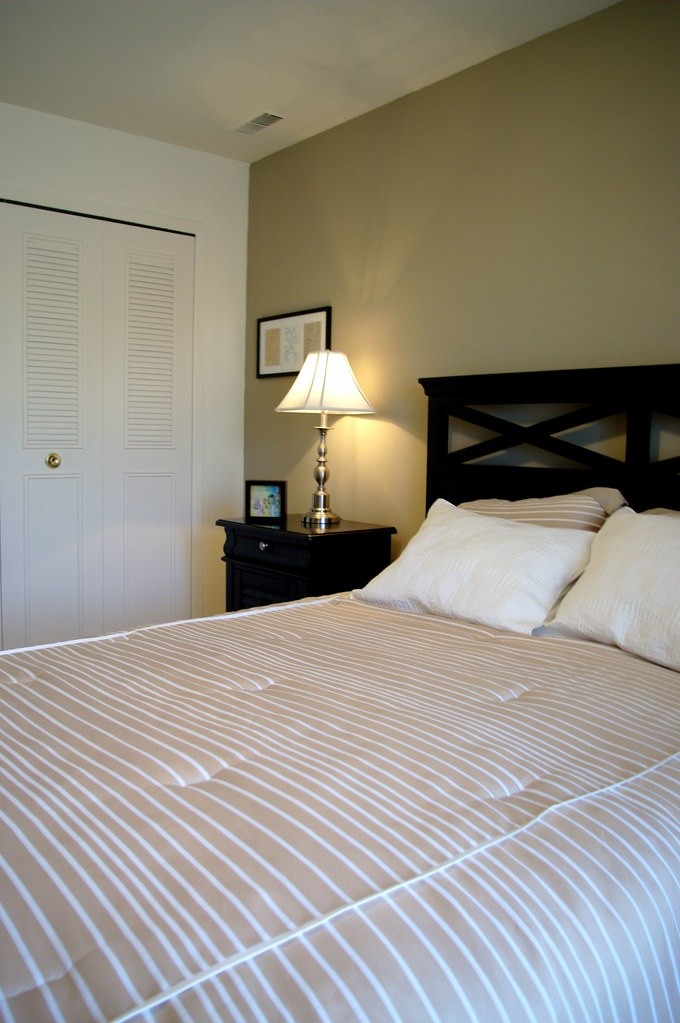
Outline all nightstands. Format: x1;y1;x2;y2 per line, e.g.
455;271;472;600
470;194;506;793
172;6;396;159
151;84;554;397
215;513;397;613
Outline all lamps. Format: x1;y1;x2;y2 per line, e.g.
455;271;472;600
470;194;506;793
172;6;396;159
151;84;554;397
274;349;376;527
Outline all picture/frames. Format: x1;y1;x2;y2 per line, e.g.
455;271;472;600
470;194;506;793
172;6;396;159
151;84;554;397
257;305;332;378
244;480;289;526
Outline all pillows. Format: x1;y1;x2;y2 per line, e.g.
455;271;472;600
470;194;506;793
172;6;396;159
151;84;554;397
351;488;680;671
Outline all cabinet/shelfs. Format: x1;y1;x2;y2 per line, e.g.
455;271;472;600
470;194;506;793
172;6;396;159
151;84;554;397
0;201;202;650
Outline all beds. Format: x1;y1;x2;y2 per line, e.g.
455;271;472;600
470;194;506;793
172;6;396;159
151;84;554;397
0;363;680;1023
